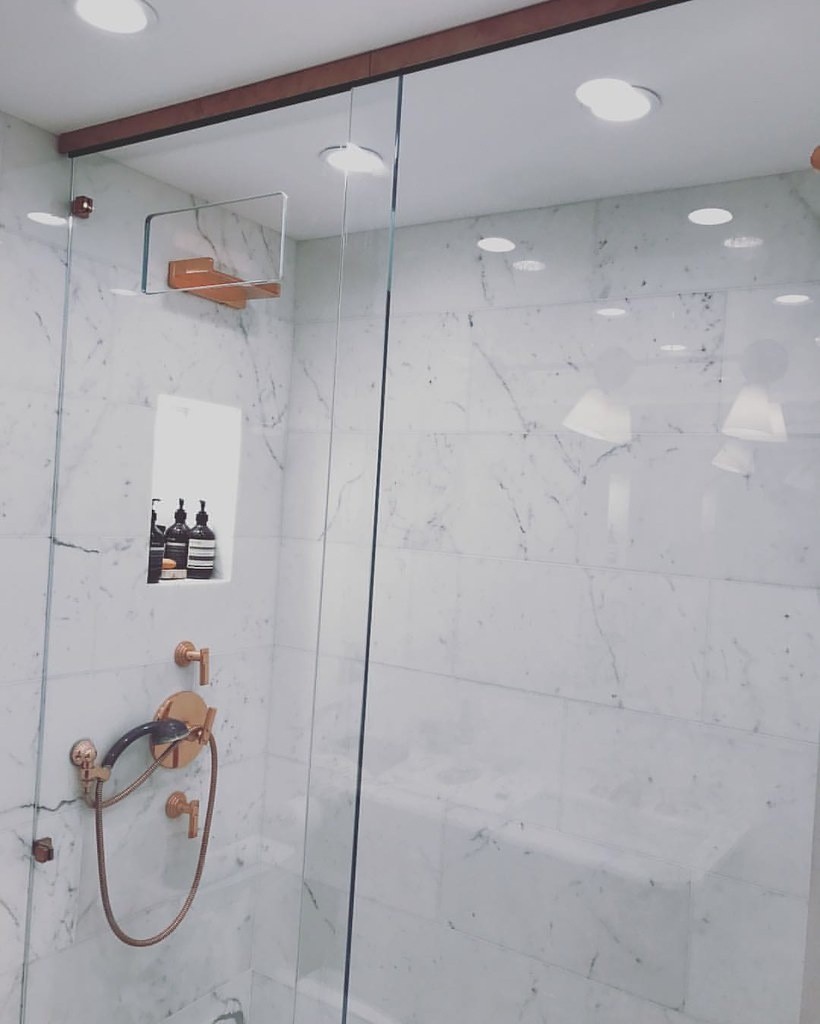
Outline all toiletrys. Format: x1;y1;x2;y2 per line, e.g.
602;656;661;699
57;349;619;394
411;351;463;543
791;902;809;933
146;498;165;584
163;497;192;572
185;498;217;580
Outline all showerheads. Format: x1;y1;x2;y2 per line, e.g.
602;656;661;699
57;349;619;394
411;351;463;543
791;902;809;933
99;717;191;771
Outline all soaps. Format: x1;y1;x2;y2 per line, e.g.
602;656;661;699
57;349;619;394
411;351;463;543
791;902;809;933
160;557;177;568
